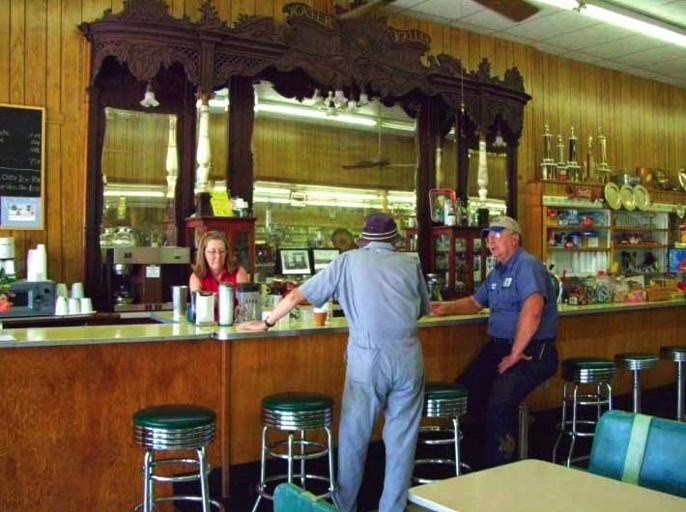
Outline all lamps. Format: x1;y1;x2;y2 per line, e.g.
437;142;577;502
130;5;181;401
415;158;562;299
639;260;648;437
139;79;161;109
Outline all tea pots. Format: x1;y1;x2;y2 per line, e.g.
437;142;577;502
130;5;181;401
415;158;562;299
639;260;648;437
234;282;262;323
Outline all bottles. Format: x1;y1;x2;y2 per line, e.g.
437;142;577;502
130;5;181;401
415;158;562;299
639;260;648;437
567;288;579;306
217;282;235;326
614;215;639;227
99;196;142;248
194;192;213;216
441;198;462;227
426;280;442;316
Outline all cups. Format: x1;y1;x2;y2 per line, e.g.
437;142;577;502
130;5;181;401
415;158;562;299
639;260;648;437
53;281;93;316
26;243;47;283
570;250;610;273
312;308;328;327
270;294;282;306
171;286;189;315
261;284;272;295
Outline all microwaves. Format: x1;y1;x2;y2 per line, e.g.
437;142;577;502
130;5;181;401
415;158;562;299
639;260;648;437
0;279;52;318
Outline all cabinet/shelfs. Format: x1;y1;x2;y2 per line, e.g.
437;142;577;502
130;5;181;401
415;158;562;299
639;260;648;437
431;224;491;300
524;178;686;293
184;216;257;287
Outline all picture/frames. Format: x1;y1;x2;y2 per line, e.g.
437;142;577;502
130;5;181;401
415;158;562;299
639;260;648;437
311;248;340;274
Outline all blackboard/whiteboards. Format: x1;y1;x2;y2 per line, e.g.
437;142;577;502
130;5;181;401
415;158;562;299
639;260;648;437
0;103;46;232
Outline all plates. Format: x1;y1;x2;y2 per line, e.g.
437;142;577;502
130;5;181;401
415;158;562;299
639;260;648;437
620;185;636;211
634;184;650;212
604;182;622;211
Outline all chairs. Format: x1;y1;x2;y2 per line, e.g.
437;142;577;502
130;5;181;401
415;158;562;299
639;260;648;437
588;409;686;500
271;482;346;512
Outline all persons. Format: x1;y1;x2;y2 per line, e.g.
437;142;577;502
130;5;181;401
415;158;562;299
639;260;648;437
432;215;558;469
237;212;434;512
185;230;253;320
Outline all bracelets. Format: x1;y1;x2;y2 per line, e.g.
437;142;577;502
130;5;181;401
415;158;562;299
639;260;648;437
262;315;274;330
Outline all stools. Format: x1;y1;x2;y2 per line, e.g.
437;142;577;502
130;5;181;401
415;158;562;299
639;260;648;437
409;381;473;484
517;402;528;459
551;356;617;467
132;404;227;512
659;345;686;423
614;353;659;414
251;391;336;512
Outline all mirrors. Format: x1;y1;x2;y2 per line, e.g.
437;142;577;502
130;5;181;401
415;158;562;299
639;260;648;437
77;21;532;302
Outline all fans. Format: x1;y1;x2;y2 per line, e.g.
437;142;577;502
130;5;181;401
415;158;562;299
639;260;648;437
341;103;416;171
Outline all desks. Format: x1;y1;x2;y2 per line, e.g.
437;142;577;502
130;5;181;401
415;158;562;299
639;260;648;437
408;459;686;512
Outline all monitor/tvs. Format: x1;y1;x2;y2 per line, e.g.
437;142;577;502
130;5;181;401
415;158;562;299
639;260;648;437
309;248;341;277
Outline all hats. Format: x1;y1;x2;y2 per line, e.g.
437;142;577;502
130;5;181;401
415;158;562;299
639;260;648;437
480;215;522;242
360;213;397;241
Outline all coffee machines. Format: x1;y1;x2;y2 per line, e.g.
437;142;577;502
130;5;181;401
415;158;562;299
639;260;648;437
101;243;193;313
